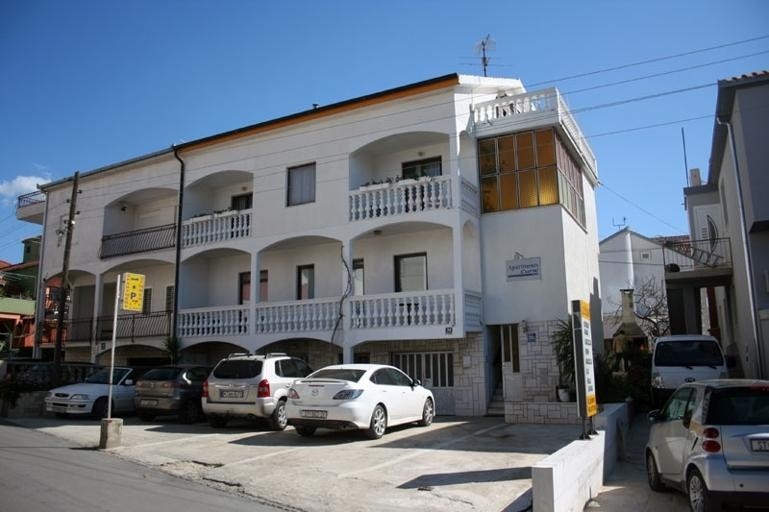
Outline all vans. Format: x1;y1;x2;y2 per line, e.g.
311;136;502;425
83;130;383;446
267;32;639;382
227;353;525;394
651;335;728;409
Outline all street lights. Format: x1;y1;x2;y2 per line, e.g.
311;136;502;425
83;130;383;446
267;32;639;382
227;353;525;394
54;172;82;364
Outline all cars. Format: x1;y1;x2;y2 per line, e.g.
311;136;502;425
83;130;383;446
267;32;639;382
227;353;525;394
646;379;769;512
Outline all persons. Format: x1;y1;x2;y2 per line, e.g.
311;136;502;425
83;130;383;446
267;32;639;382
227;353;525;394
613;329;630;372
